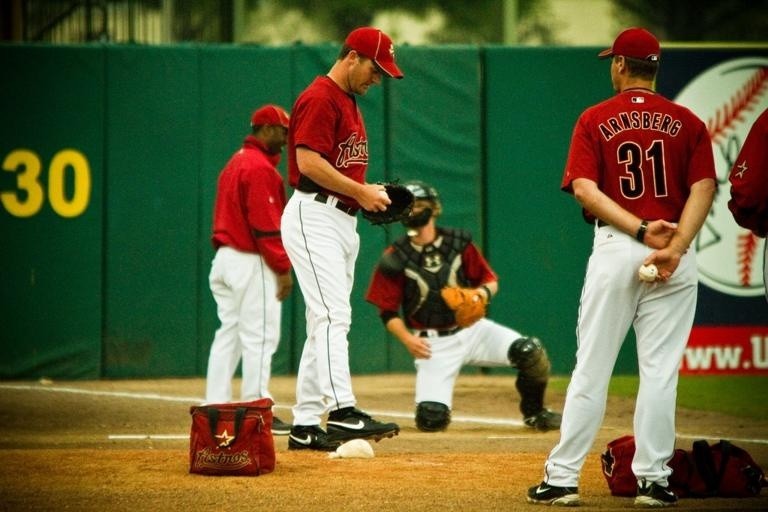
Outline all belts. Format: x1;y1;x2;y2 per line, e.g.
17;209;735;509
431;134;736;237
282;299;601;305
311;192;360;217
418;327;466;338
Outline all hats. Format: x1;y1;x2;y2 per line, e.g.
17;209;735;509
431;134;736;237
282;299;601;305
249;102;293;130
343;25;406;81
596;25;663;66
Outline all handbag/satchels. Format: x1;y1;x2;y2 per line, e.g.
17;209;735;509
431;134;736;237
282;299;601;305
183;398;281;475
598;433;768;503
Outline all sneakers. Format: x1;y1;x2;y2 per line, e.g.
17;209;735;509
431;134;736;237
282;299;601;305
632;478;682;508
525;479;582;507
522;408;564;431
326;407;400;446
269;414;295;436
287;423;346;452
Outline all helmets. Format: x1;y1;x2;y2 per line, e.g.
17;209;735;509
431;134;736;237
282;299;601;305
398;179;441;227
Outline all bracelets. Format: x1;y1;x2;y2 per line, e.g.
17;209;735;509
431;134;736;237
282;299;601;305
637;220;648;244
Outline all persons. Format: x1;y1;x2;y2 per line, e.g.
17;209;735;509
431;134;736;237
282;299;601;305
364;181;562;431
527;28;718;507
280;26;404;451
728;108;768;305
206;104;295;436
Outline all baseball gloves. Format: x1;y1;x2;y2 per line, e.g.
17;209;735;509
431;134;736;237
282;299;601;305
440;287;488;327
360;181;415;226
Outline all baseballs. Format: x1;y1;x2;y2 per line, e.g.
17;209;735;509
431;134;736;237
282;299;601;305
639;263;658;281
380;191;389;199
